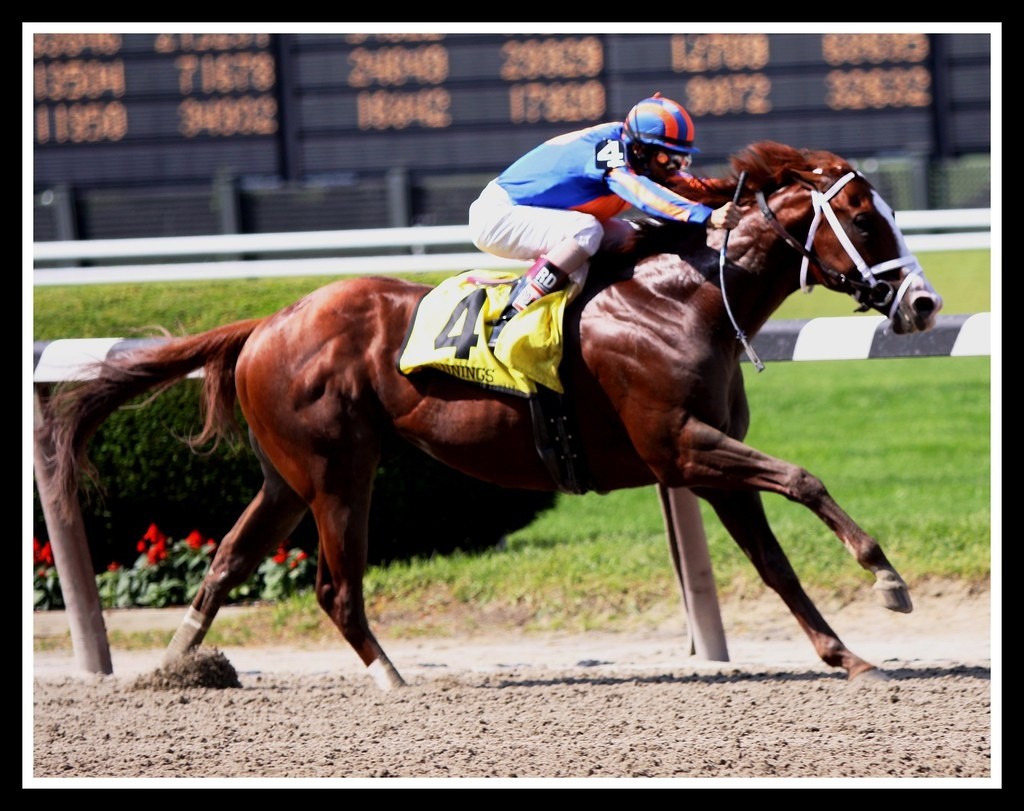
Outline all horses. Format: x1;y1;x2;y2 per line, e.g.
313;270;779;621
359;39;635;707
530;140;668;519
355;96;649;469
40;141;945;700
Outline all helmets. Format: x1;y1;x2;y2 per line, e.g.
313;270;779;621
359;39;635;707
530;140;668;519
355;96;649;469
622;92;700;153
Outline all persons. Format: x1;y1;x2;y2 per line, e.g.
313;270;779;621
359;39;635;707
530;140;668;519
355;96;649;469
468;91;750;346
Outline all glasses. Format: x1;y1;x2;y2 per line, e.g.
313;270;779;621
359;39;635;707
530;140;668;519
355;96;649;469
640;143;692;170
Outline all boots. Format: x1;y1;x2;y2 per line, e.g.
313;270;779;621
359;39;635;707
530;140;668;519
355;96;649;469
485;257;568;350
